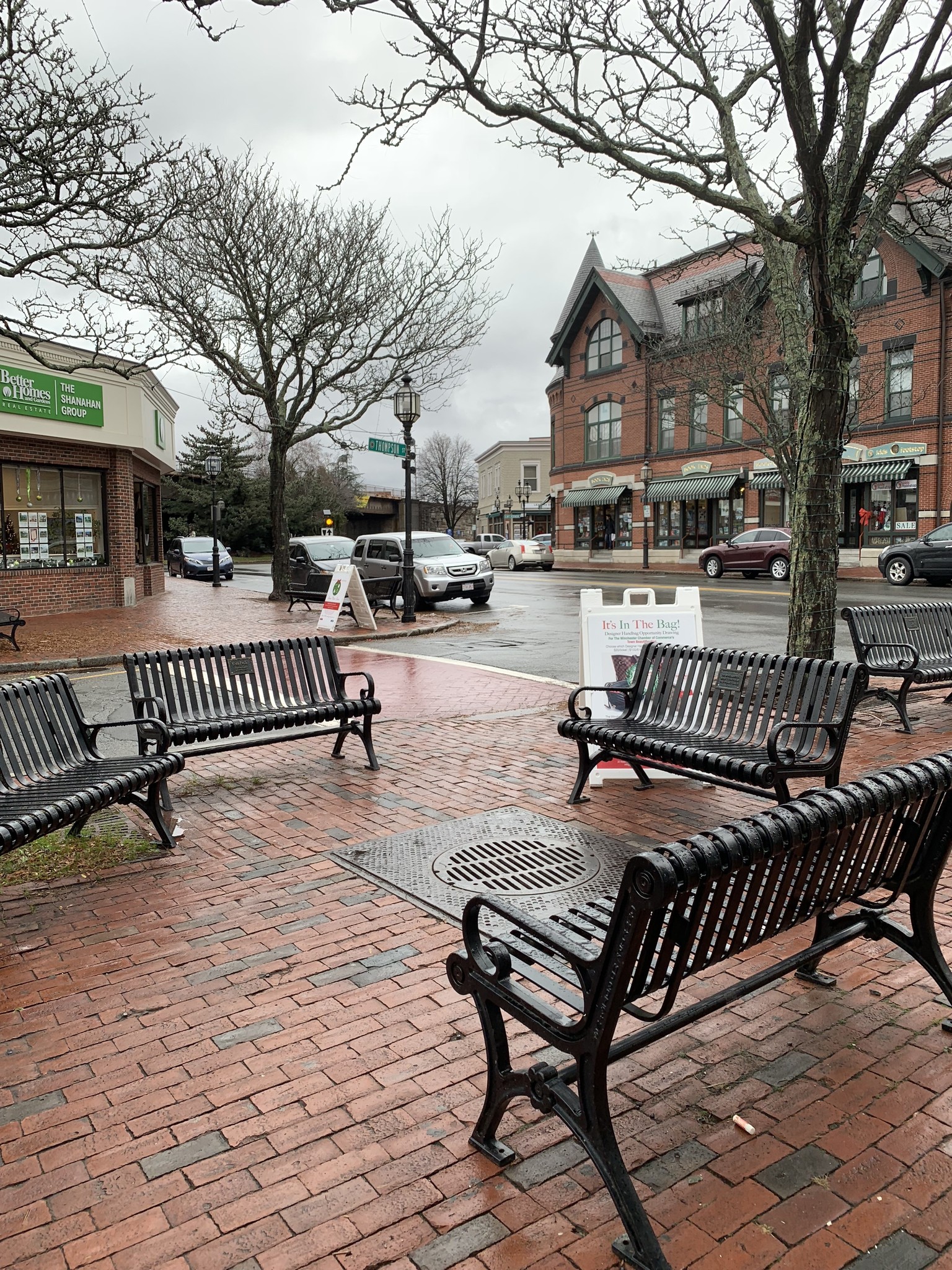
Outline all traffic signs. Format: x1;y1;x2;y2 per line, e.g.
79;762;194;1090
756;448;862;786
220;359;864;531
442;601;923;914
368;437;407;458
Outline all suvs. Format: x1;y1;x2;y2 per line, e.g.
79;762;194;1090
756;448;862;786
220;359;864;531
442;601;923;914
878;521;952;586
271;536;356;592
167;536;234;580
351;531;495;611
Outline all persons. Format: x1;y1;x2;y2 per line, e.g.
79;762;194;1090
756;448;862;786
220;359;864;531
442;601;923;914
603;514;615;550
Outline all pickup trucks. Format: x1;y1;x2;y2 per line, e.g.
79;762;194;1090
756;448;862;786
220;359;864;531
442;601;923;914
459;533;508;555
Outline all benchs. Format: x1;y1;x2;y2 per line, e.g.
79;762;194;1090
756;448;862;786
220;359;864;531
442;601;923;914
121;634;384;811
0;672;186;887
0;605;26;652
840;597;951;735
445;747;952;1269
554;641;869;852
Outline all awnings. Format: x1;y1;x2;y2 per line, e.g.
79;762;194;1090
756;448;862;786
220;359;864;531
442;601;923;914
640;473;740;504
538;494;552;511
747;472;793;491
838;458;914;484
560;485;627;509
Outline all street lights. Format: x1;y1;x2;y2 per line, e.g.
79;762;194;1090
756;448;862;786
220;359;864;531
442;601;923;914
204;446;226;586
494;495;513;536
515;480;532;540
394;370;421;624
639;459;652;569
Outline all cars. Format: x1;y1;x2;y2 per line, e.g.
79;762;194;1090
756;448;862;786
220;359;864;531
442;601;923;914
486;539;555;571
532;533;552;547
698;527;793;581
